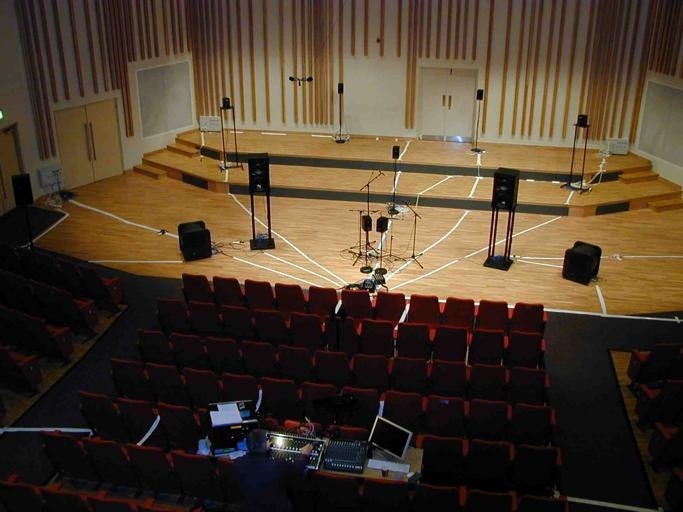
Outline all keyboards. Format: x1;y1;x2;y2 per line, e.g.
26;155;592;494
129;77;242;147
367;458;411;473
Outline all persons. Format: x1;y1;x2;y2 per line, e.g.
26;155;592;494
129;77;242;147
234;428;313;512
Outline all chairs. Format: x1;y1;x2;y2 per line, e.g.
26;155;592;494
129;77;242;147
2;246;569;512
625;341;683;512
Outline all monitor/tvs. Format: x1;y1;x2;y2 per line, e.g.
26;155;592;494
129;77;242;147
368;415;414;461
208;399;258;428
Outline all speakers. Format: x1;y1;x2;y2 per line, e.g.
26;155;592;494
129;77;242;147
247;153;271;193
491;167;520;211
392;146;399;159
376;217;388;232
223;97;231;108
12;173;34;206
361;216;372;231
338;83;343;94
477;89;483;100
578;115;587;126
562;240;602;285
177;220;212;261
